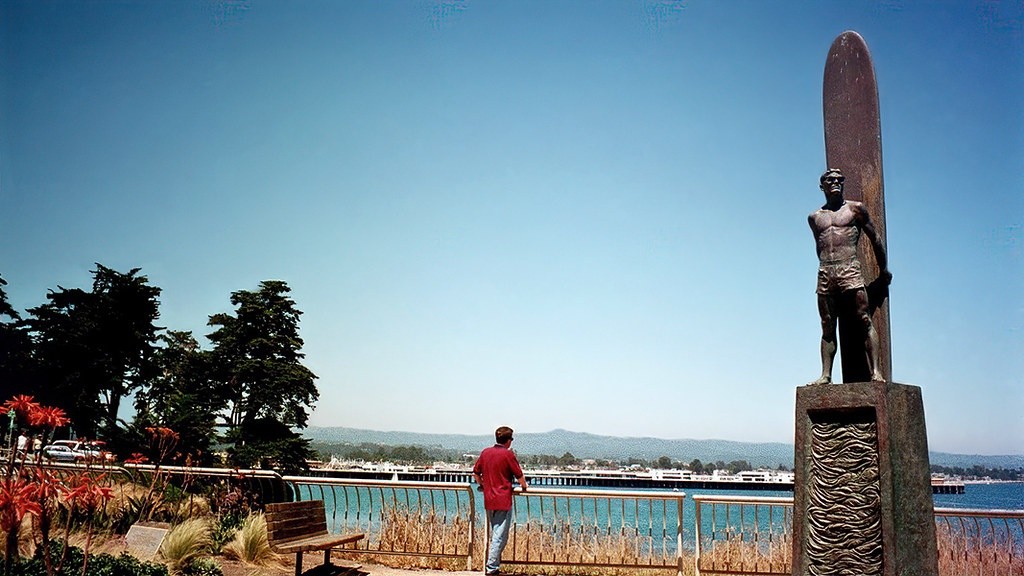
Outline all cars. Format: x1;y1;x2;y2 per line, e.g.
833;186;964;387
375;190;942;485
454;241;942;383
0;449;32;462
38;445;85;464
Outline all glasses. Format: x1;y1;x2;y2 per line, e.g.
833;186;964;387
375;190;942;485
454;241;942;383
511;438;514;441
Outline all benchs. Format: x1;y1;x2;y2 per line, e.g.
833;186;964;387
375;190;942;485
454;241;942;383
264;499;365;576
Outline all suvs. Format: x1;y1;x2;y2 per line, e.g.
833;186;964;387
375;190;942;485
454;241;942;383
52;439;112;463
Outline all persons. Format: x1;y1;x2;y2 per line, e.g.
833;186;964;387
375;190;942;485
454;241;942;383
473;426;529;576
17;432;27;459
33;434;43;461
807;169;893;385
